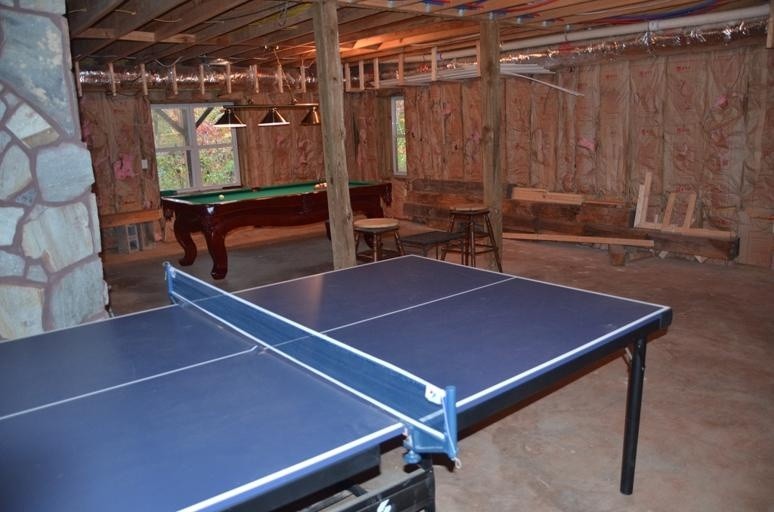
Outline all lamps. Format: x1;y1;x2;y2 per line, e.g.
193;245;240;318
211;104;320;129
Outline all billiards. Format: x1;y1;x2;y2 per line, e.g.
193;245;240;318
219;193;225;200
314;182;327;189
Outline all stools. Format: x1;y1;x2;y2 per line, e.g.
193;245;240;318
439;203;504;273
352;218;405;262
397;229;465;265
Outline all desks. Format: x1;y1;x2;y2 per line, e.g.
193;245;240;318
158;179;393;281
0;251;675;510
